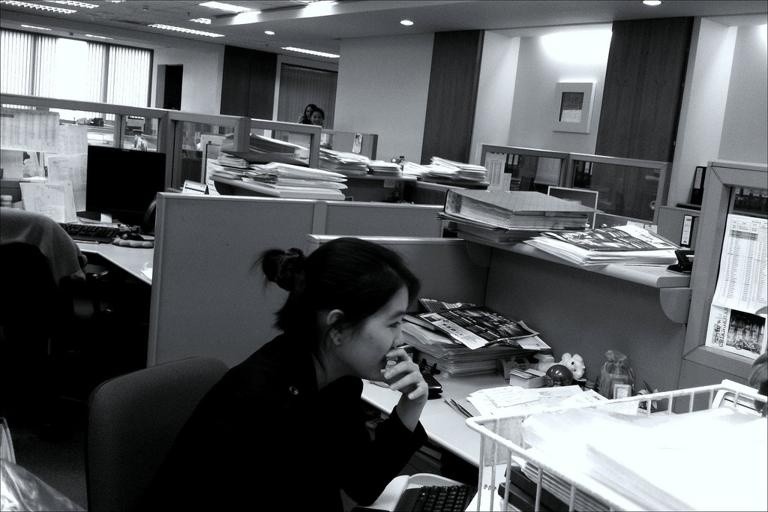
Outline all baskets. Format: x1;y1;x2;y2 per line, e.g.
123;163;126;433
465;381;767;512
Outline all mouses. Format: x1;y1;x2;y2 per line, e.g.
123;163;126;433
122;230;144;242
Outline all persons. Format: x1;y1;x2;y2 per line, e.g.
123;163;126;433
298;104;328;129
167;237;429;512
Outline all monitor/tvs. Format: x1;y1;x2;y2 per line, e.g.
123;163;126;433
547;185;599;230
87;144;166;226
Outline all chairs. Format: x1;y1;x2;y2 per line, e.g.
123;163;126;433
1;214;121;444
89;358;231;512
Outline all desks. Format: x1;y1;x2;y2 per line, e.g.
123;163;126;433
310;161;768;512
1;94;445;370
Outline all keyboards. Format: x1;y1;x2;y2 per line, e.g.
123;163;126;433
391;484;477;512
54;222;118;243
